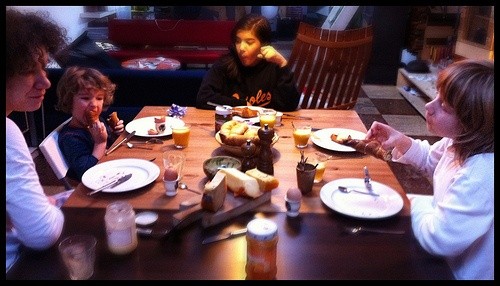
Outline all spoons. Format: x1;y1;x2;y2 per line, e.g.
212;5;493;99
338;185;380;198
178;182;201;195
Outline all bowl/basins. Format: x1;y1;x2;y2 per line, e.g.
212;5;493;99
203;155;241;180
215;126;279;155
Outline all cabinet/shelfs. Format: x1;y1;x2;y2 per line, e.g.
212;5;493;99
396;6;494;121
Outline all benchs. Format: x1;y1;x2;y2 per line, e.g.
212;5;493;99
34;17;238;125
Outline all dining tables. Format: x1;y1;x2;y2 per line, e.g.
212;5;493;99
61;106;414;218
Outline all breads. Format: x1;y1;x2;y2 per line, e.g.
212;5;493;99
201;171;227;213
339;135;393;159
219;167;263;198
232;106;259;118
220;121;260;147
86;111;98;127
245;168;278;192
106;112;119;129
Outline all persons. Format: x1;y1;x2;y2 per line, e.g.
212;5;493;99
365;61;495;280
5;9;64;273
197;14;300;111
58;66;124;180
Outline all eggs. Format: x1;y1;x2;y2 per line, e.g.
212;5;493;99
155;116;165;122
287;187;302;200
164;167;178;181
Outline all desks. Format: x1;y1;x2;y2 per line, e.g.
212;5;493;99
121;57;181;70
81;11;115;23
6;224;457;280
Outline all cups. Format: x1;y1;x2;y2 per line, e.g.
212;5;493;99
292;122;311;148
59;234;97;280
162;120;192;197
285;157;330;217
257;108;283;129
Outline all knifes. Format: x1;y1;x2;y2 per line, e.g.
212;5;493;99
201;228;247;244
87;174;132;196
105;131;136;155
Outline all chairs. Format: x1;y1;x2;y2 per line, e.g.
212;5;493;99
286;23;374;110
39;116;73;191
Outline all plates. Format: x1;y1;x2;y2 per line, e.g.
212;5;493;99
312;128;368;151
81;158;160;193
125;116;185;137
230;105;263;125
320;178;404;218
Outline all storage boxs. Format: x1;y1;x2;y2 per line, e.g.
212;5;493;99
87;27;109;40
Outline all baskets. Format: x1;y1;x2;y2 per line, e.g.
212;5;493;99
215;126;279;158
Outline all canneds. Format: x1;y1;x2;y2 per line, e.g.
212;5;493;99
245;218;278;280
104;200;137;254
215;105;232;133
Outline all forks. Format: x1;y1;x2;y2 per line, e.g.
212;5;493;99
337;221;404;235
